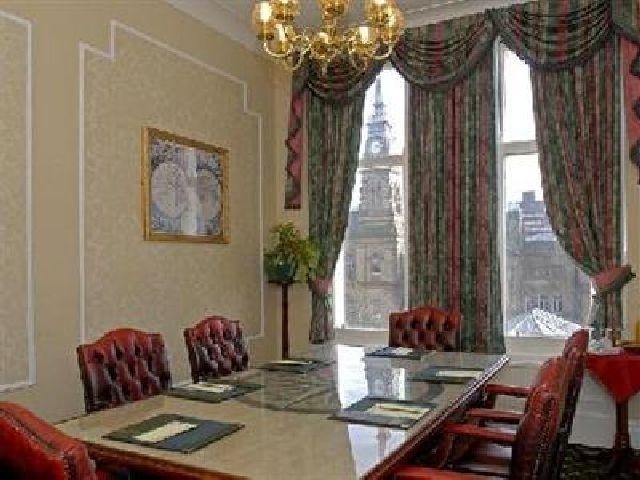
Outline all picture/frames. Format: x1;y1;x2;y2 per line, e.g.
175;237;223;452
138;126;232;247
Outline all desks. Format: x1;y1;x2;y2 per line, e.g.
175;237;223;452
53;347;510;479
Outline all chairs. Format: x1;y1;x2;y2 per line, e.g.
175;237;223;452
77;328;172;414
2;401;129;480
390;311;460;352
389;356;570;480
464;328;587;475
184;315;249;379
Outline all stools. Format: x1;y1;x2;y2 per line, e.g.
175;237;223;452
585;352;640;477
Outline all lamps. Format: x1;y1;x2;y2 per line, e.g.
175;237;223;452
250;1;407;75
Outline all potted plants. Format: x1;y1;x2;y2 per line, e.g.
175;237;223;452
263;219;321;283
